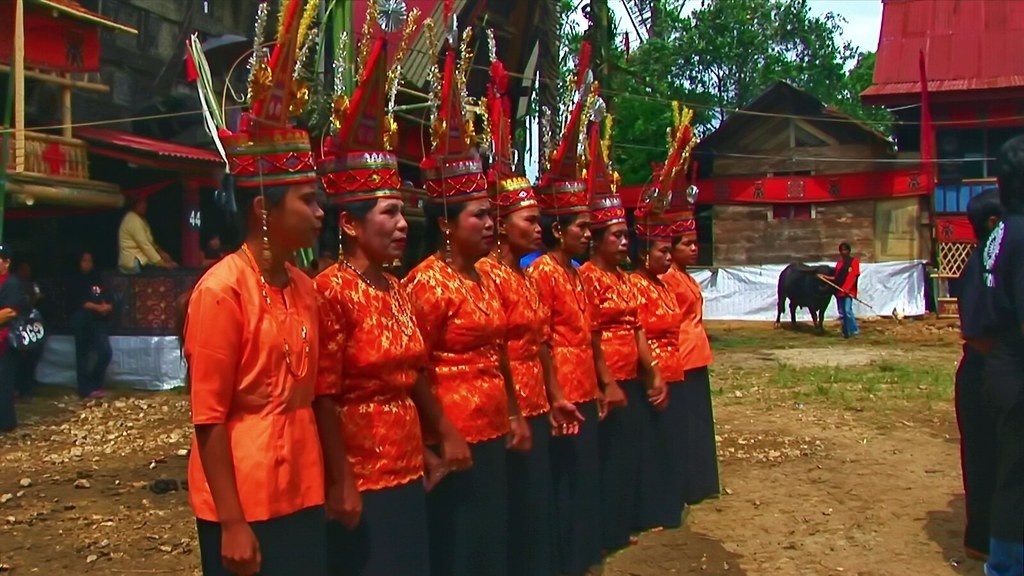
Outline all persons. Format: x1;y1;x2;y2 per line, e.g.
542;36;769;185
0;242;49;434
400;157;719;576
117;187;177;275
954;133;1024;576
822;241;860;339
311;149;430;576
71;248;113;399
202;230;225;259
178;128;364;576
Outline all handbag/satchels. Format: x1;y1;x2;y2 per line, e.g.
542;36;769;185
9;307;51;353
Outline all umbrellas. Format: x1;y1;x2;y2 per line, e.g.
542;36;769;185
182;33;251;102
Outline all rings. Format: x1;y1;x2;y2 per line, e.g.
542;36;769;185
435;473;442;477
450;465;457;470
563;423;567;427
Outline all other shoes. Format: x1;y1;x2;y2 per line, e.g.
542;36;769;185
963;533;991;561
87;389;109;399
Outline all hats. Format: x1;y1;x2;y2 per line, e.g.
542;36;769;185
307;0;423;204
483;28;538;217
184;0;336;186
418;0;488;204
633;100;696;241
536;36;591;214
0;245;13;259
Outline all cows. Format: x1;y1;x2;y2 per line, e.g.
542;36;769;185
773;261;837;338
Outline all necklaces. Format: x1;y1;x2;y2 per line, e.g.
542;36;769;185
437;248;491;315
491;250;539;309
240;244;311;380
590;255;632;305
549;251;587;313
671;264;701;300
634;271;676;311
340;259;414;336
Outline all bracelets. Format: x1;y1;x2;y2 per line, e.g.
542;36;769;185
650;359;659;366
509;415;520;420
